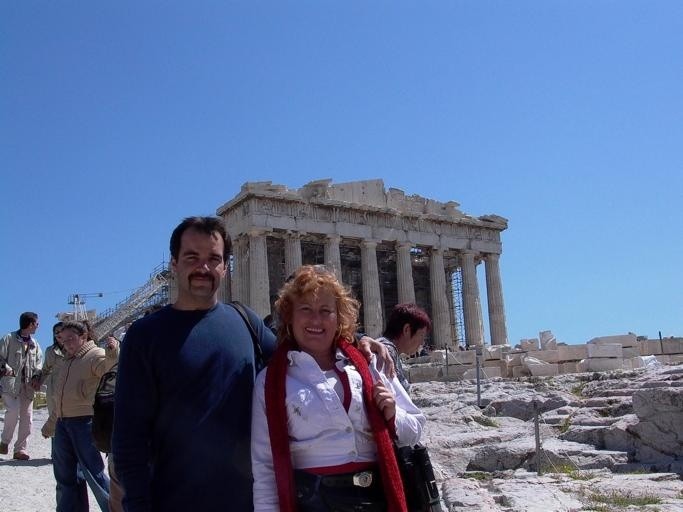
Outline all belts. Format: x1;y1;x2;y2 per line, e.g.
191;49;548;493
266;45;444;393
320;471;381;490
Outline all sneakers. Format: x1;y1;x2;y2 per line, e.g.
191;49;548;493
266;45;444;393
13;452;29;460
0;442;8;454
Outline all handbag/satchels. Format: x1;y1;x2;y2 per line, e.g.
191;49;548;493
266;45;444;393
395;441;442;512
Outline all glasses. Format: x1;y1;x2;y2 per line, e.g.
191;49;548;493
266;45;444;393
61;333;83;345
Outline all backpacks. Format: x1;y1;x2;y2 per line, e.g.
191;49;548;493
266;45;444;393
93;363;118;453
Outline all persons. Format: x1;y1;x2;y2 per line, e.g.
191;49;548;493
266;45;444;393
367;301;432;403
104;297;166;512
32;321;71;418
108;216;398;511
0;310;44;460
39;319;122;511
243;264;426;512
